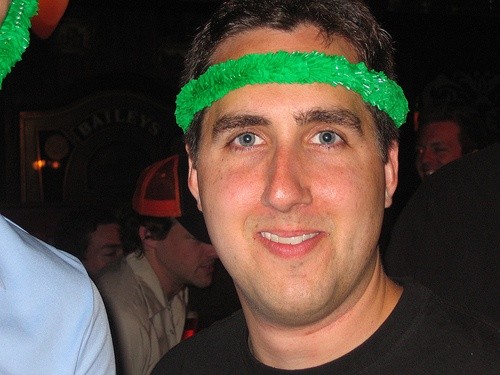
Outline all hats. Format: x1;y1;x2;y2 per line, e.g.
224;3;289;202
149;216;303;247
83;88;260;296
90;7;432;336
131;152;212;244
